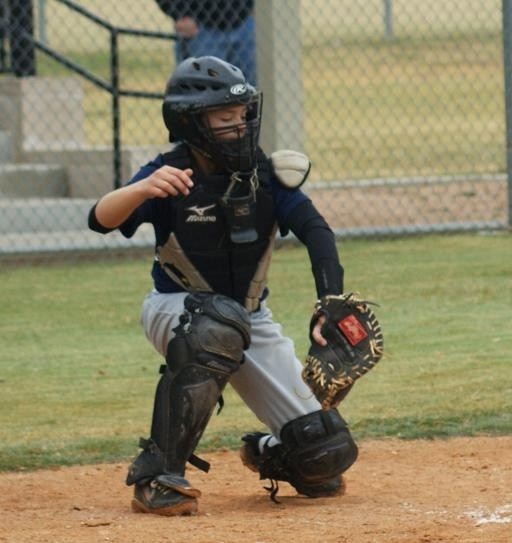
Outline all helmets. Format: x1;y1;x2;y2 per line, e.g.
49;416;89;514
162;54;263;172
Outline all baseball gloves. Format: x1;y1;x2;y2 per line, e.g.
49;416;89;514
302;292;385;410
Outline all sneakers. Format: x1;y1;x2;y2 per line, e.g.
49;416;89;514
132;476;198;519
239;432;346;499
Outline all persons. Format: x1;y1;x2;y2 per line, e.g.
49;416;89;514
83;54;385;518
152;0;260;90
